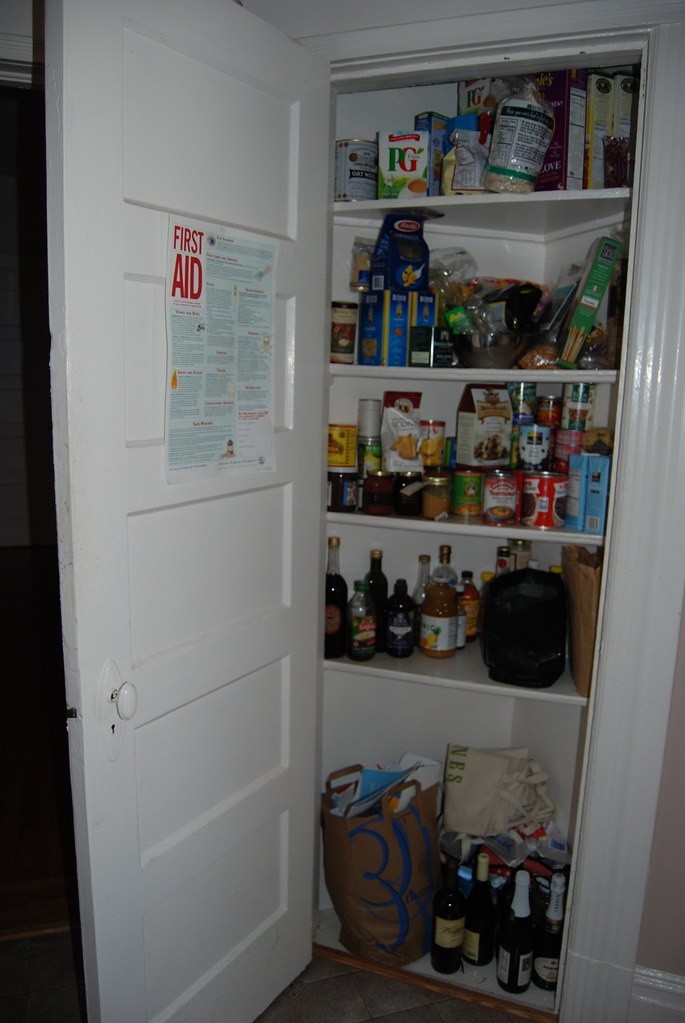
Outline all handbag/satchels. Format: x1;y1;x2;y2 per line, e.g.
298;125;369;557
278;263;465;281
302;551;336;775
320;763;445;967
560;544;601;696
441;742;557;837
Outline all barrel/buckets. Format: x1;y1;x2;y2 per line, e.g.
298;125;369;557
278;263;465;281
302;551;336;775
335;139;378;202
508;381;597;473
484;471;569;529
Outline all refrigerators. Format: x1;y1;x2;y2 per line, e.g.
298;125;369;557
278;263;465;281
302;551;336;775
46;0;685;1023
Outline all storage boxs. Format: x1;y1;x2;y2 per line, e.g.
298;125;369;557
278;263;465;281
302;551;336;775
375;130;429;198
354;288;413;367
408;326;454;369
557;236;624;370
458;78;491;117
583;74;615;190
612;74;640;187
529;69;588;193
411;292;439;327
414;111;452;197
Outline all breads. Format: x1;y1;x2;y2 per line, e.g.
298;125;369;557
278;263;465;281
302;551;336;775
518;345;559;369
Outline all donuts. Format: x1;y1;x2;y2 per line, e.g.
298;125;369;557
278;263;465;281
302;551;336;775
581;426;615;455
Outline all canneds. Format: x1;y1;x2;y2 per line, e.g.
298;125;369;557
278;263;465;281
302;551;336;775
334;138;380;201
329;382;597;530
331;300;359;364
508;539;531;571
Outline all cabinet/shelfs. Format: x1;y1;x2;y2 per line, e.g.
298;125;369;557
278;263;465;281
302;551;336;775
309;16;662;1023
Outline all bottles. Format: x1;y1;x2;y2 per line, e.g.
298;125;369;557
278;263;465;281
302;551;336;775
464;853;497;967
461;570;479;643
496;870;534;994
432;868;465;975
363;466;450;519
414;555;432;645
421;544;466;660
331;302;358;364
532;873;566;991
365;550;388;654
454;469;482;515
324;536;348;660
387;579;415;659
481;538;564;596
327;424;357;513
348;581;375;662
